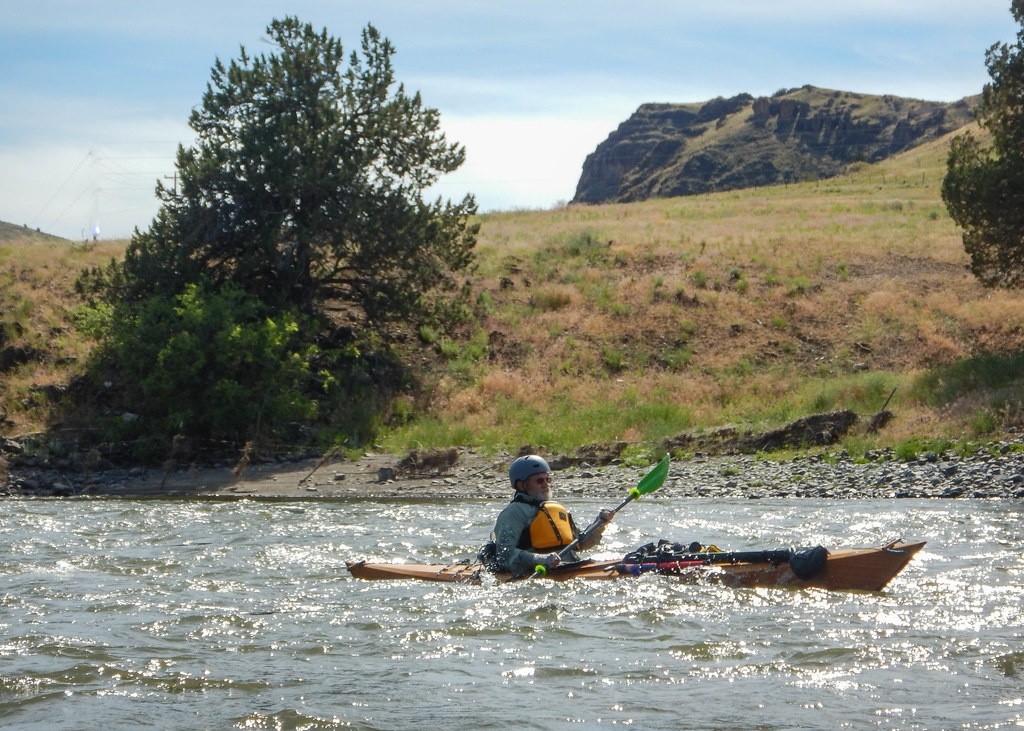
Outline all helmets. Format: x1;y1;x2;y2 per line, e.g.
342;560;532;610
509;455;551;489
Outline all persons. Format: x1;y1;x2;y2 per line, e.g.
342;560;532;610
493;454;616;574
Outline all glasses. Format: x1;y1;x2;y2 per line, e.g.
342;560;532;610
529;478;551;484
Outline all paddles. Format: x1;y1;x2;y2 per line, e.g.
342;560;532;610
527;451;671;580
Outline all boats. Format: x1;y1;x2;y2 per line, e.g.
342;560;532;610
344;538;928;596
